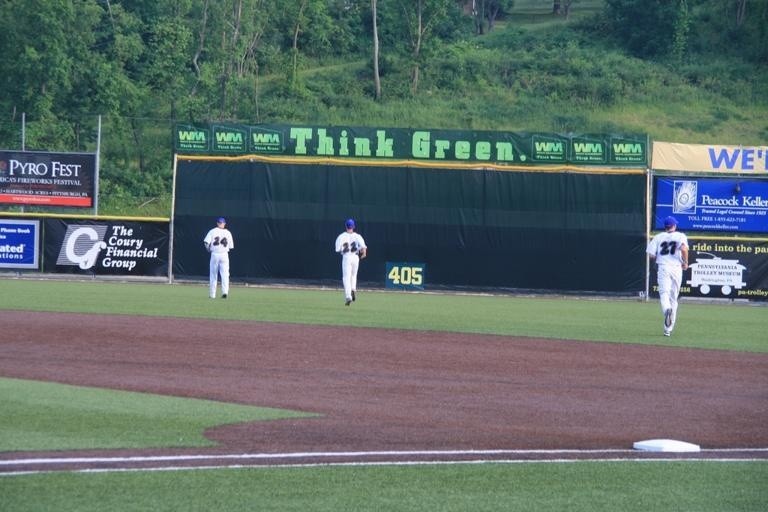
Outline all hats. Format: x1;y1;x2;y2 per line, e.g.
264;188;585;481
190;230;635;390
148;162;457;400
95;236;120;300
664;216;680;225
217;217;226;224
346;218;356;229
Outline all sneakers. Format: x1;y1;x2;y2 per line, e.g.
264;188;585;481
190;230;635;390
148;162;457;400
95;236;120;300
664;308;672;336
209;294;227;299
345;290;356;306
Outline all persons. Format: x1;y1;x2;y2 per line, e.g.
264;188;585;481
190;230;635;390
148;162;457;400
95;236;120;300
334;218;368;306
646;216;689;336
203;217;234;300
